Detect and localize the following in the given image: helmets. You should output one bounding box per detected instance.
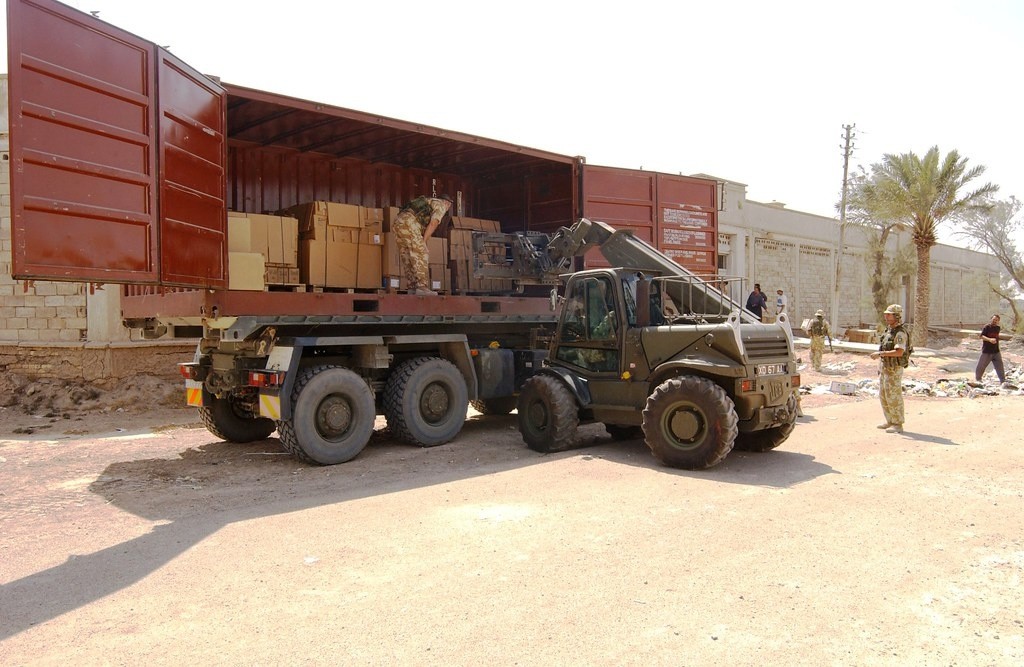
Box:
[883,304,903,314]
[814,309,826,317]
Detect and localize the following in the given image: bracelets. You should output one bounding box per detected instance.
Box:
[878,351,883,357]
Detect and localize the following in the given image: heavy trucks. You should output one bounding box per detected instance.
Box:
[6,0,719,466]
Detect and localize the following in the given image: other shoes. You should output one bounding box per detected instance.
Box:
[877,422,892,429]
[886,423,903,433]
[1001,379,1013,385]
[408,288,416,295]
[416,286,438,296]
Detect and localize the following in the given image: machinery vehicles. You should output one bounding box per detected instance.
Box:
[471,217,801,470]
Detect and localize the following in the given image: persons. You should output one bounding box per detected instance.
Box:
[805,309,834,371]
[723,279,728,296]
[869,304,910,433]
[392,193,455,295]
[581,310,616,363]
[745,284,769,322]
[975,315,1007,384]
[774,288,787,322]
